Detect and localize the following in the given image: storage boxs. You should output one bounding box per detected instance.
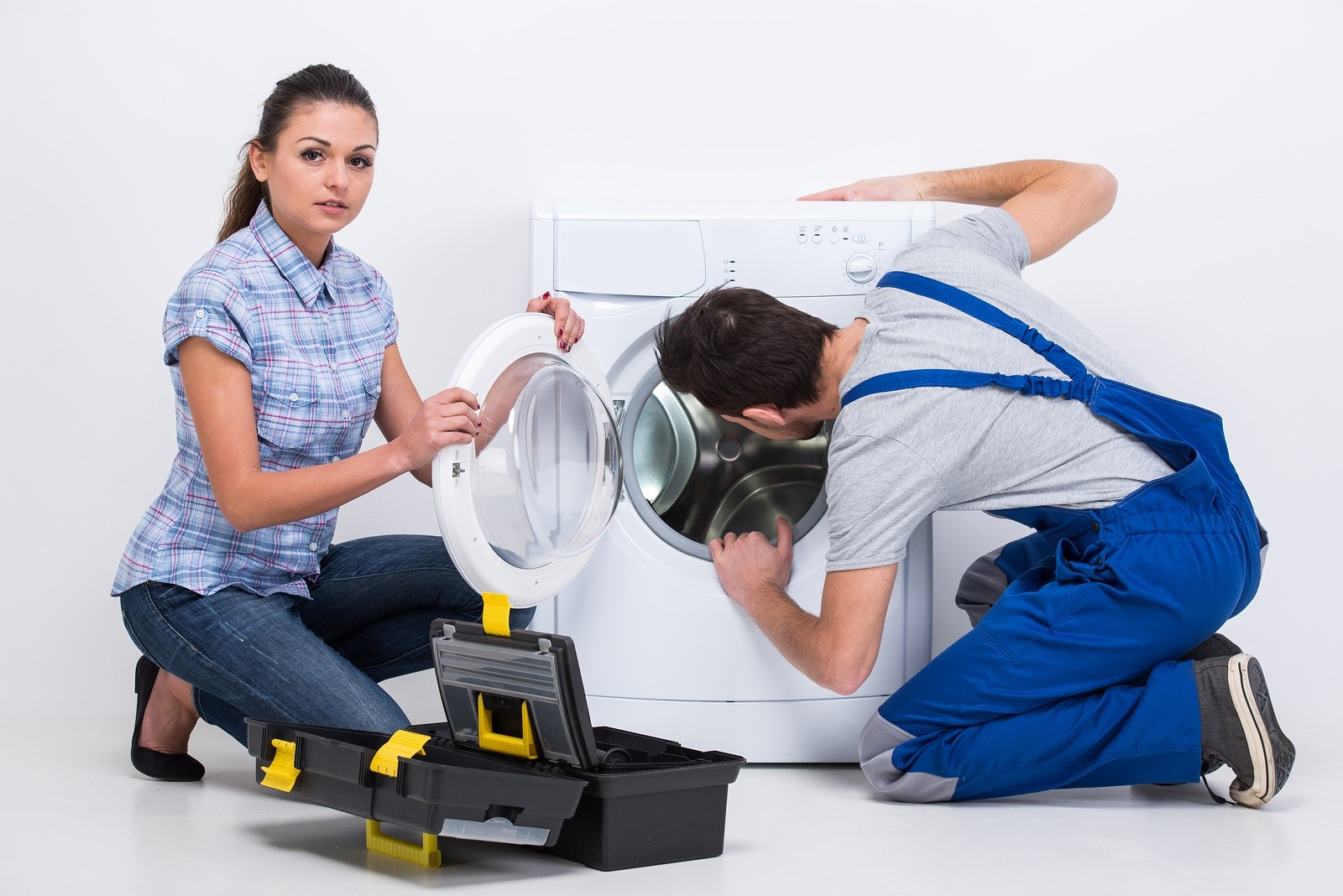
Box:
[244,591,746,872]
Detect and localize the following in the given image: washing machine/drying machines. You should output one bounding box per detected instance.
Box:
[428,204,934,770]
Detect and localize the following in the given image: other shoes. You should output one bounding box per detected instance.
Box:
[130,652,206,783]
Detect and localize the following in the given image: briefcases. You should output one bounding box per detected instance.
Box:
[243,615,747,872]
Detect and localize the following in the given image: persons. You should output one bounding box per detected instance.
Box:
[653,157,1295,808]
[112,66,584,787]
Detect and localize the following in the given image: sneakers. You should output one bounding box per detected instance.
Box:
[1196,633,1295,807]
